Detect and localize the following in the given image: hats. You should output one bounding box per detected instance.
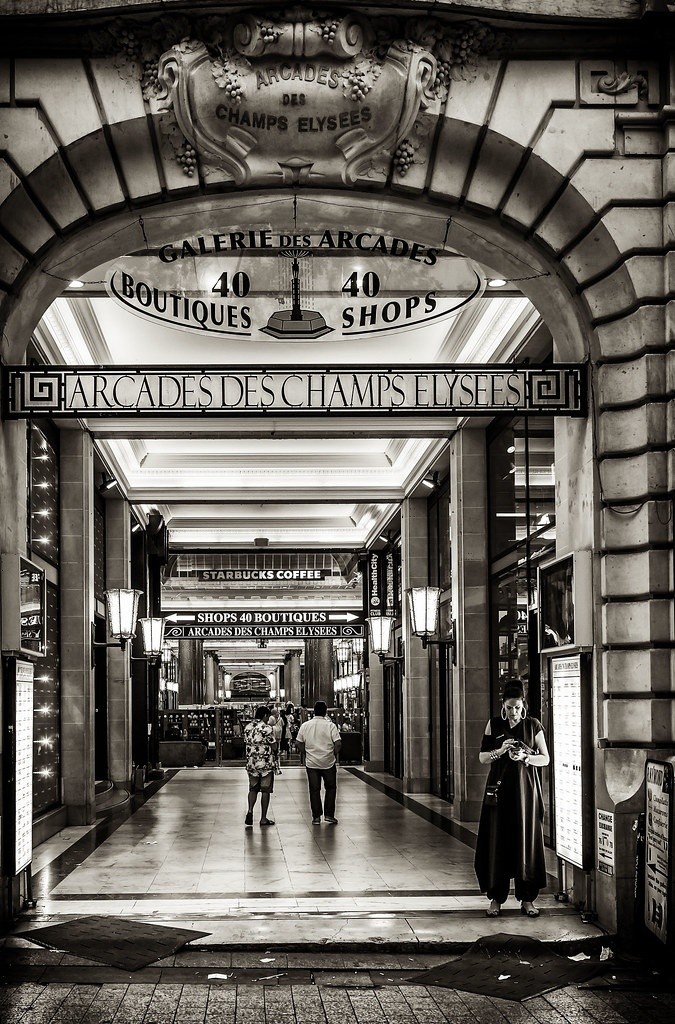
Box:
[501,680,524,699]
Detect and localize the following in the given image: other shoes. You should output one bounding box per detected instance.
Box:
[487,905,500,917]
[521,902,540,918]
[312,817,321,825]
[324,816,338,824]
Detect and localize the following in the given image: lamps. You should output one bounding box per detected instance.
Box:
[91,589,145,670]
[130,617,168,678]
[404,586,457,667]
[365,616,405,677]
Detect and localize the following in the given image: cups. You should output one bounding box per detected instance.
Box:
[156,761,161,770]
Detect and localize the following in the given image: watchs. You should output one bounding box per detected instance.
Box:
[524,753,530,764]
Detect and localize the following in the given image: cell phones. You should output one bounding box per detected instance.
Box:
[512,741,519,752]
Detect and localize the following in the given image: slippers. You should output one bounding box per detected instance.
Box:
[245,811,253,825]
[260,818,274,826]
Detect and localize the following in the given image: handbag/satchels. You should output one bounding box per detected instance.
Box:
[515,741,537,767]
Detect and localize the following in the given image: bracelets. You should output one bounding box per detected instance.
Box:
[489,749,501,761]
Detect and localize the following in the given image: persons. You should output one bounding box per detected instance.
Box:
[475,681,551,917]
[268,703,296,760]
[295,701,343,825]
[243,706,279,825]
